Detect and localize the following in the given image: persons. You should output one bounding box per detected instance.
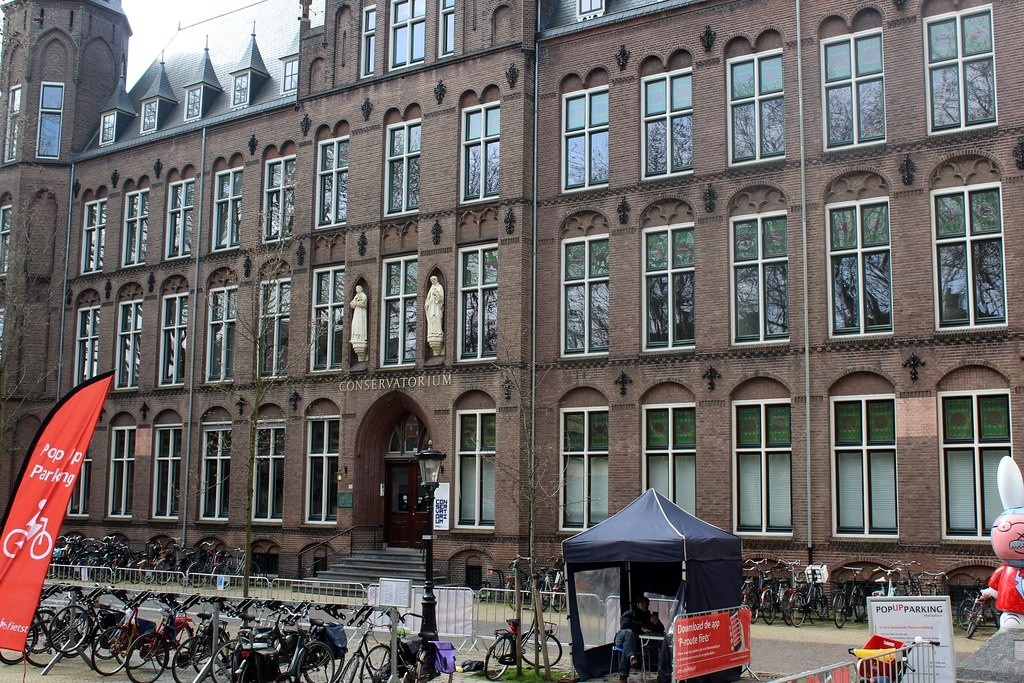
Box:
[349,284,370,344]
[613,597,666,683]
[424,275,445,338]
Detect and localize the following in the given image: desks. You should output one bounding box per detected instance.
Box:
[639,633,664,683]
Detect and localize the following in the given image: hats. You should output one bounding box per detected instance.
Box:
[636,597,650,604]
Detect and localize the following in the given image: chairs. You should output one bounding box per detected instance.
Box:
[610,646,650,678]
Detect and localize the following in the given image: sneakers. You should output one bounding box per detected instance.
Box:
[619,677,627,683]
[629,655,639,669]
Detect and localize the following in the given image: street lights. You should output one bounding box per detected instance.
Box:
[414,439,447,642]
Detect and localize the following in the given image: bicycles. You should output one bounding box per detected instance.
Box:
[0,582,453,683]
[849,638,940,683]
[485,608,562,680]
[741,558,949,629]
[958,576,990,639]
[505,553,566,612]
[47,536,259,589]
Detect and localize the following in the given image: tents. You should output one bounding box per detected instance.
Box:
[561,488,744,683]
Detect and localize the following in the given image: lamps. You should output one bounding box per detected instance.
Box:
[335,466,346,482]
